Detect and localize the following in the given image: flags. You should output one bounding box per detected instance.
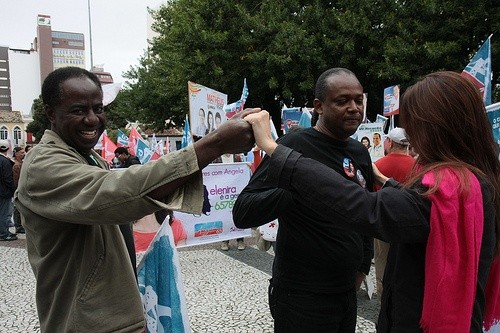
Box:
[482,102,500,151]
[100,114,195,166]
[226,80,249,118]
[460,37,492,106]
[298,110,312,128]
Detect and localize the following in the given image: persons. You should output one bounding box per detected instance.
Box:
[114,146,141,167]
[215,151,246,250]
[132,207,187,254]
[244,70,500,333]
[205,110,215,135]
[192,107,206,137]
[360,127,420,282]
[214,111,223,130]
[230,65,377,333]
[389,85,399,112]
[0,145,32,241]
[12,65,264,333]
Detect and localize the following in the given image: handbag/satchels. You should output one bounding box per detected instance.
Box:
[254,227,273,251]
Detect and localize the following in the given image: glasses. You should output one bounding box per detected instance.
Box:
[116,154,121,159]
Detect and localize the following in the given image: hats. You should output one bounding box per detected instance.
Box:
[13,147,23,157]
[0,140,9,151]
[385,127,409,145]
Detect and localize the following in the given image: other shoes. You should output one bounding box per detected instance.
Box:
[238,242,245,249]
[0,231,18,241]
[16,226,25,233]
[222,240,229,251]
